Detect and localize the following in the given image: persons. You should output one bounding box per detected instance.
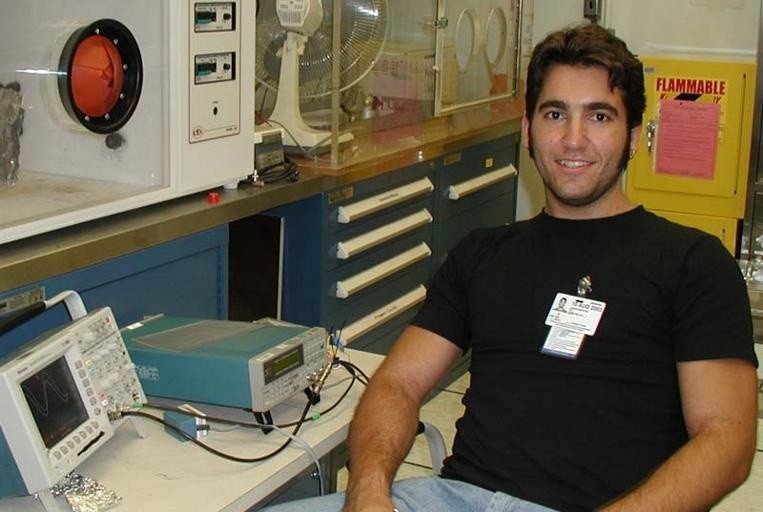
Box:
[554,297,569,312]
[256,20,762,512]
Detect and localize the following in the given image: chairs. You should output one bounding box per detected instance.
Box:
[346,421,448,486]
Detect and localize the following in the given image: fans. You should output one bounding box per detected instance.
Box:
[255,0,389,157]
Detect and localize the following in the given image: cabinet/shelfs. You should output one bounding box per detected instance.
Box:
[320,128,521,349]
[627,45,757,259]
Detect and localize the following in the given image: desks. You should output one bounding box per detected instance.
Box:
[0,347,390,512]
